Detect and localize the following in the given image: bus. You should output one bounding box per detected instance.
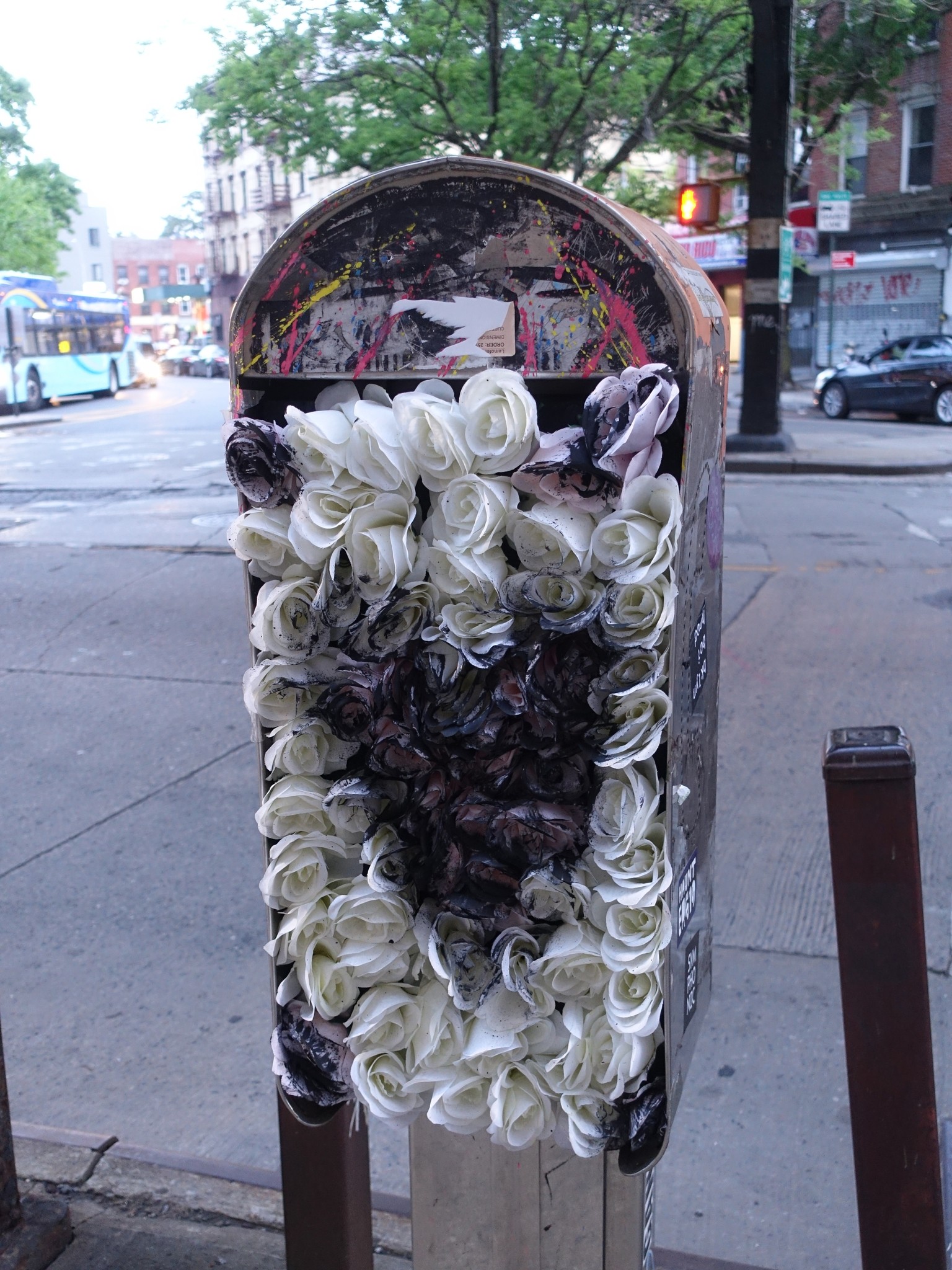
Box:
[0,272,137,413]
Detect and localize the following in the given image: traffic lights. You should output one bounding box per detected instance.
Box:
[676,180,721,226]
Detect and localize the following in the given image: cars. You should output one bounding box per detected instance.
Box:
[155,345,201,375]
[812,334,952,427]
[189,344,229,376]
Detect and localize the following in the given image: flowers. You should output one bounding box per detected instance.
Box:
[210,351,697,1160]
